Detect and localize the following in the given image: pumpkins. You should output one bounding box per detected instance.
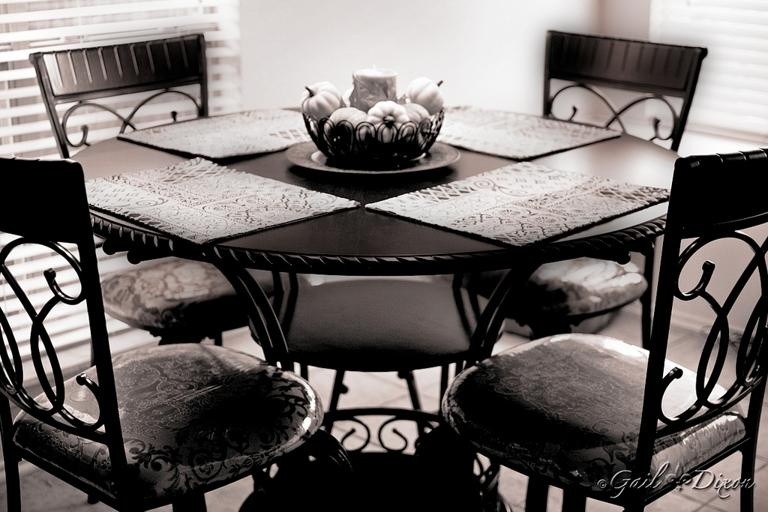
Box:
[301,78,444,149]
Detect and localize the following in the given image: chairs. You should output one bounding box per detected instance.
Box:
[0,31,768,512]
